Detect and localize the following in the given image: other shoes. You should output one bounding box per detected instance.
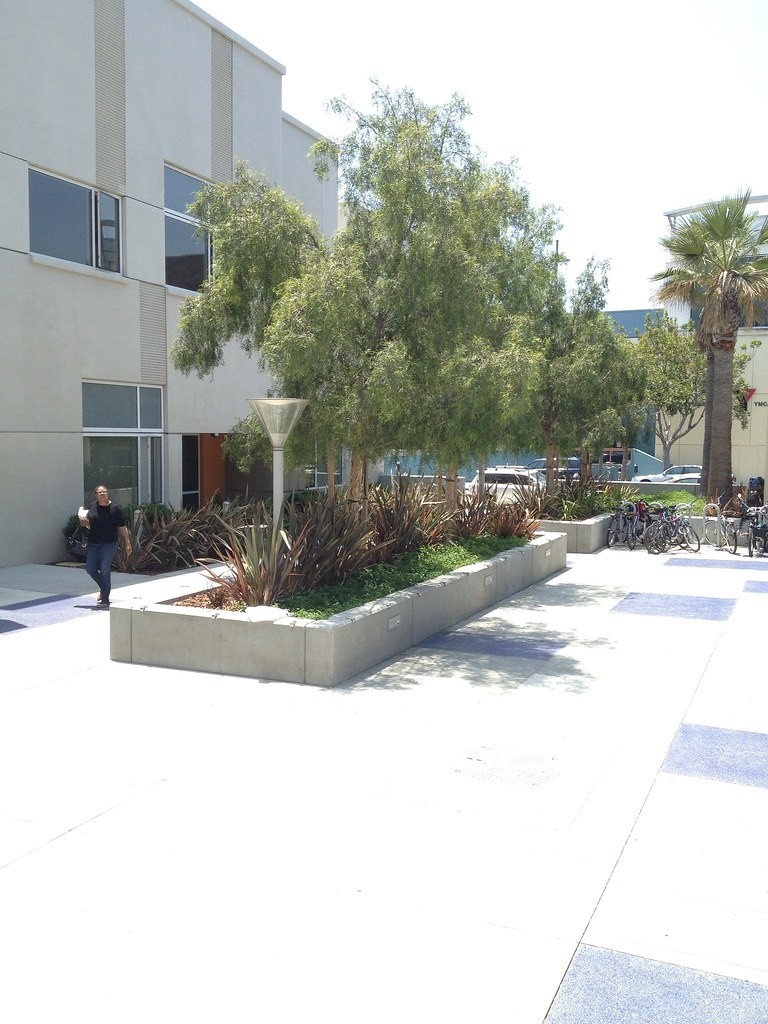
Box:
[97,603,110,608]
[97,591,102,601]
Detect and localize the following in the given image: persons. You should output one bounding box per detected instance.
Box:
[80,485,132,608]
[746,477,765,507]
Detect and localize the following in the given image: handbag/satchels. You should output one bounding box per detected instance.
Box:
[66,526,89,556]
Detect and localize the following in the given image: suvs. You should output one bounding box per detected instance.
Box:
[467,465,548,507]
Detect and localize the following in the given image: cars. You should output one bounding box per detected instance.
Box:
[526,457,583,479]
[605,500,768,558]
[631,464,737,484]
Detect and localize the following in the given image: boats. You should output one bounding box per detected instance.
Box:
[247,396,310,556]
